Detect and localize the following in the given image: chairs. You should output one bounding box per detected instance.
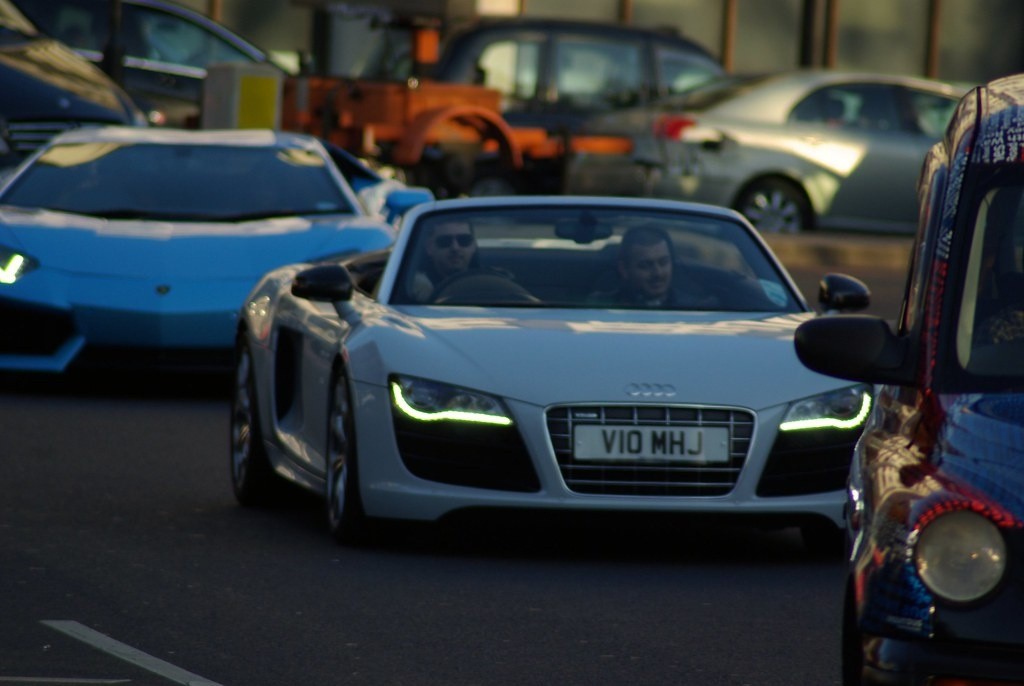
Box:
[471,242,774,310]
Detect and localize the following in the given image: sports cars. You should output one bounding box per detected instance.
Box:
[229,196,878,555]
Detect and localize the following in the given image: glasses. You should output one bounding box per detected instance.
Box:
[431,233,475,248]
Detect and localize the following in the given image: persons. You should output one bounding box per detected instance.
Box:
[584,228,718,312]
[408,216,530,304]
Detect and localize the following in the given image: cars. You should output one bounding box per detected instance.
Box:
[0,123,435,374]
[353,17,748,197]
[15,0,302,122]
[1,0,153,174]
[565,66,985,240]
[792,73,1024,686]
